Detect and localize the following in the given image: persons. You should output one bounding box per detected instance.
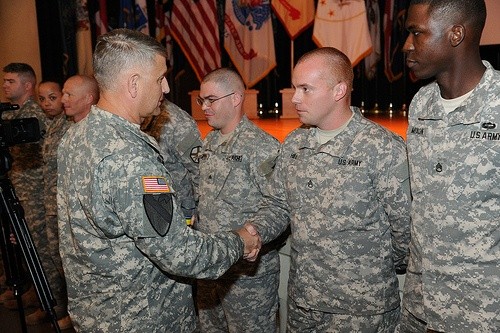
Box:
[191,67,281,333]
[142,98,202,229]
[56,28,261,333]
[0,62,99,333]
[244,47,412,333]
[398,0,500,333]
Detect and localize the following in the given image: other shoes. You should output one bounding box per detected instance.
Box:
[0,275,72,329]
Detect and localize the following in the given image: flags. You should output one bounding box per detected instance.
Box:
[73,0,422,95]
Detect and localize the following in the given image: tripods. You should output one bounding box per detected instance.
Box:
[0,149,61,333]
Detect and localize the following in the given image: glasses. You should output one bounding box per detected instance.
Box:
[196,92,234,106]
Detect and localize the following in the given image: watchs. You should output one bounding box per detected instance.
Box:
[228,231,244,252]
